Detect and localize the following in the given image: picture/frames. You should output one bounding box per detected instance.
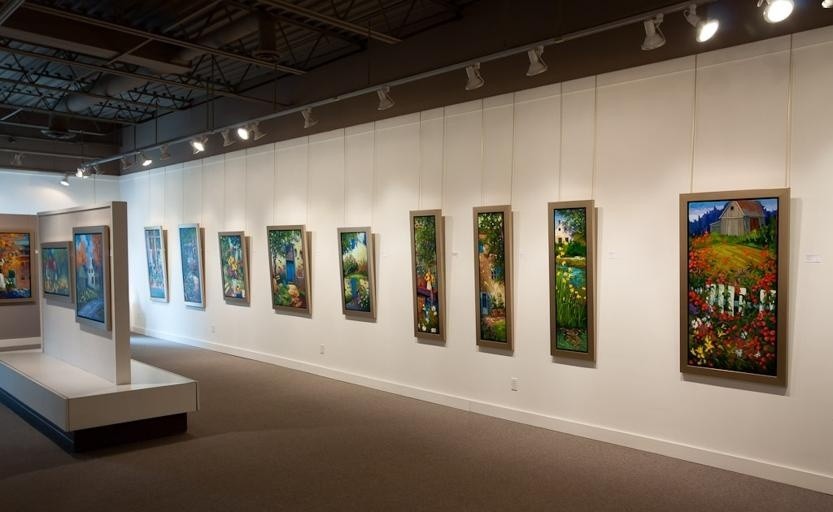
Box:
[143,225,377,320]
[409,200,598,362]
[0,225,109,330]
[681,186,790,388]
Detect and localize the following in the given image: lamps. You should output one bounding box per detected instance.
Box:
[640,0,833,54]
[60,140,172,190]
[187,118,265,155]
[377,83,394,113]
[300,105,317,131]
[459,45,549,92]
